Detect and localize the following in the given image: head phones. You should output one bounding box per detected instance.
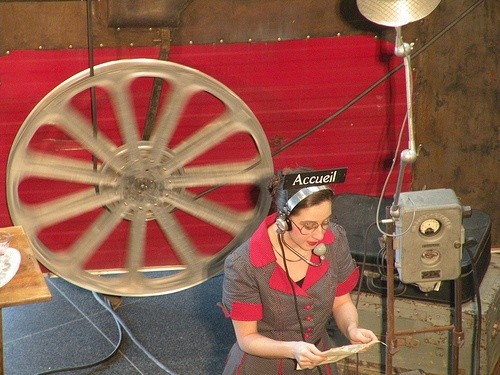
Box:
[276,184,334,234]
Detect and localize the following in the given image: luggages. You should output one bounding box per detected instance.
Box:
[331,193,492,304]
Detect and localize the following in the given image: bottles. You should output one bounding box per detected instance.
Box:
[0,230,11,268]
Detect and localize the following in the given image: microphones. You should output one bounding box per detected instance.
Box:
[281,234,326,267]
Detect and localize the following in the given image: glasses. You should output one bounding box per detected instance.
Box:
[286,216,336,235]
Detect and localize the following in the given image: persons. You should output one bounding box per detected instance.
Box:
[221,166,380,375]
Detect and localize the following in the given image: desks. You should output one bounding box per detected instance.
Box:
[0,226,52,375]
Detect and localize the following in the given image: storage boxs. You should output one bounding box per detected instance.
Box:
[326,253,500,375]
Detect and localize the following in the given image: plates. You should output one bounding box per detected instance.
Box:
[0,248,21,287]
